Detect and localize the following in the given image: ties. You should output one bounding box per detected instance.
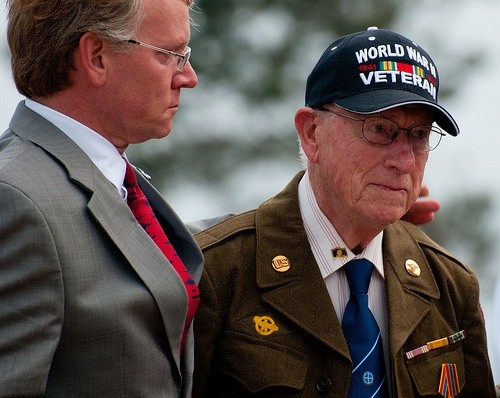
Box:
[341,259,390,398]
[123,161,200,355]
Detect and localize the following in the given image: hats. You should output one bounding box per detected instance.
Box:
[305,26,460,136]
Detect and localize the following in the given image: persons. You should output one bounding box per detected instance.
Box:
[0,1,440,398]
[191,26,498,398]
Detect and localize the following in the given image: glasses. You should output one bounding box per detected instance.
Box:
[311,105,446,152]
[124,39,192,70]
